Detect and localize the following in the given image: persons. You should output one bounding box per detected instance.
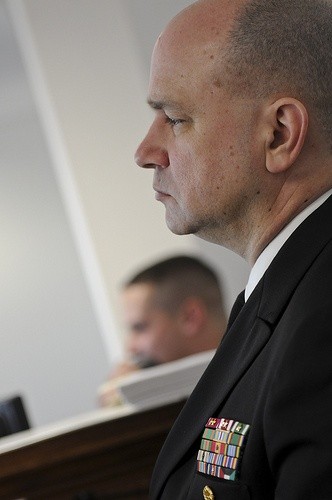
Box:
[121,254,230,366]
[130,0,331,499]
